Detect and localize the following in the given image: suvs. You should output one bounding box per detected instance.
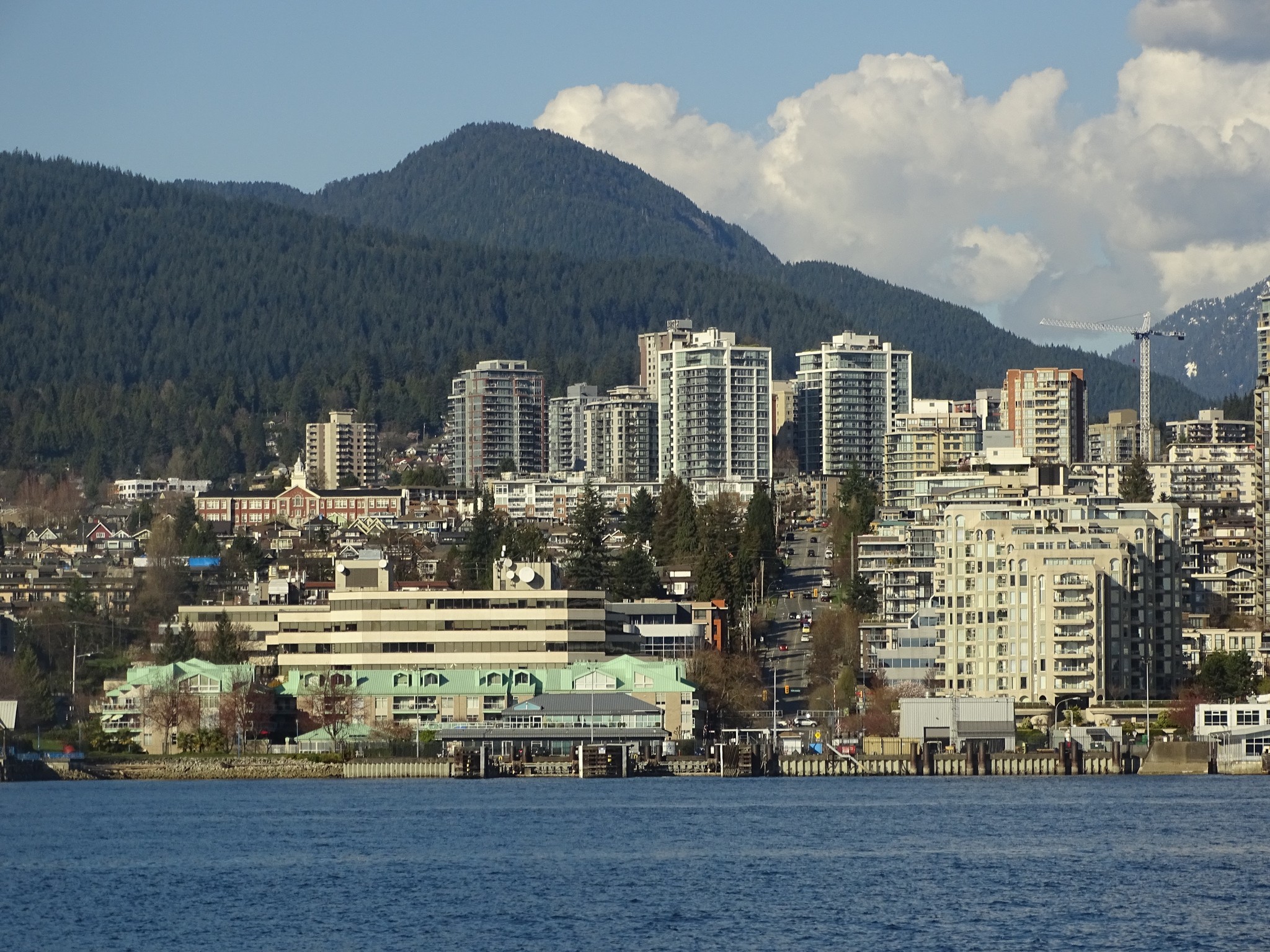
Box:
[760,717,787,728]
[535,747,549,757]
[808,550,815,557]
[811,537,817,543]
[786,533,795,541]
[794,718,817,728]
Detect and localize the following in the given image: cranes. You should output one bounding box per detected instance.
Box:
[1039,311,1185,461]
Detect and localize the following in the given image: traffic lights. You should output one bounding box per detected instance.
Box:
[785,685,789,694]
[814,589,817,597]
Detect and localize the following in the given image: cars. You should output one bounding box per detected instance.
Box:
[806,517,813,523]
[801,635,810,642]
[779,643,788,650]
[822,522,828,528]
[802,592,812,599]
[821,546,833,601]
[787,549,794,555]
[790,610,813,632]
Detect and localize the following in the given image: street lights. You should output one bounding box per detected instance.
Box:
[1140,656,1152,750]
[767,580,782,597]
[815,698,834,740]
[72,645,91,711]
[816,676,833,687]
[768,658,782,754]
[413,666,423,756]
[587,659,599,743]
[1055,697,1080,730]
